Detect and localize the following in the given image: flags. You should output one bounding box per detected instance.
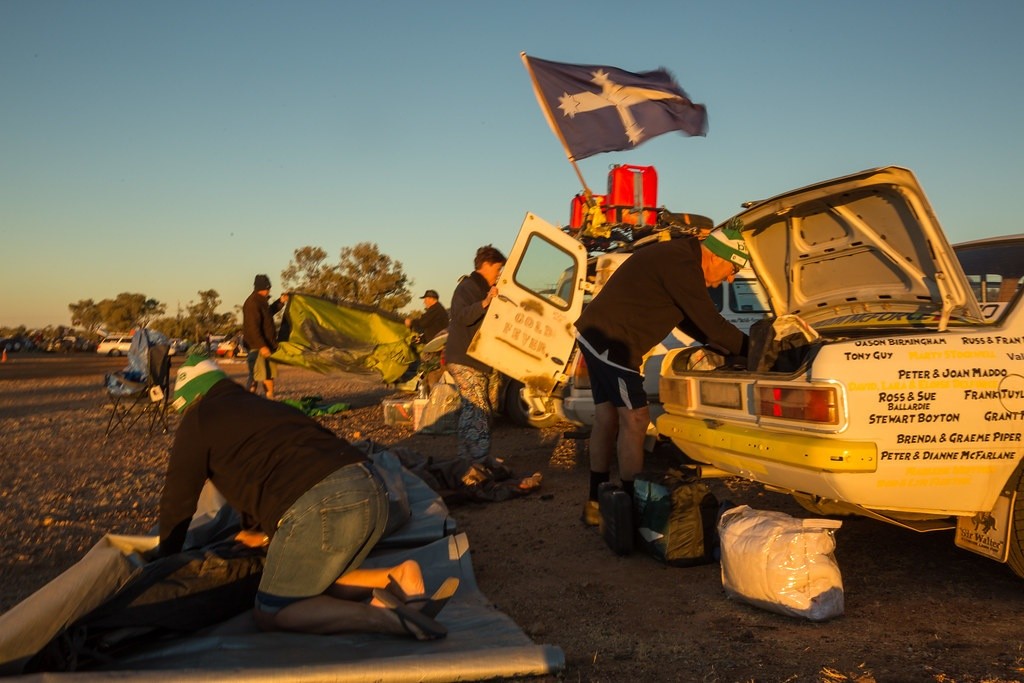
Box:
[526,56,710,160]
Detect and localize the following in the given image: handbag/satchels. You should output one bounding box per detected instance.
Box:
[636,464,719,567]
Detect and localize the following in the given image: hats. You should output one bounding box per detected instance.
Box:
[702,217,750,268]
[419,290,438,299]
[174,343,227,413]
[254,274,270,290]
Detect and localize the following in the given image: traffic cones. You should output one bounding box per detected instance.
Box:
[0,348,9,363]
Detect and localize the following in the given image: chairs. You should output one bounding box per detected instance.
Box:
[103,329,171,438]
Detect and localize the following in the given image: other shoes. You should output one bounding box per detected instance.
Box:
[584,502,603,525]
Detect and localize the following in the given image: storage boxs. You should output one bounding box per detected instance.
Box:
[606,165,657,226]
[382,399,414,427]
[413,399,429,430]
[570,194,608,228]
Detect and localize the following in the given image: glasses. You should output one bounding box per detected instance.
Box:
[733,263,739,274]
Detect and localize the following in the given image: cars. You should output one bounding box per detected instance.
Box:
[464,208,775,466]
[215,329,250,359]
[0,338,26,352]
[94,328,182,357]
[652,163,1024,589]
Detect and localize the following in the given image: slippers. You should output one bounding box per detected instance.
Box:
[373,589,447,639]
[404,576,460,618]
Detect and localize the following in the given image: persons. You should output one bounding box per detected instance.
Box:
[571,227,752,529]
[16,324,243,357]
[242,274,288,398]
[147,355,460,641]
[405,290,450,392]
[443,242,505,459]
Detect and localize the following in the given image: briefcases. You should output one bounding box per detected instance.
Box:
[598,482,633,555]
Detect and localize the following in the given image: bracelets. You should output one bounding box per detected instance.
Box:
[261,534,269,545]
